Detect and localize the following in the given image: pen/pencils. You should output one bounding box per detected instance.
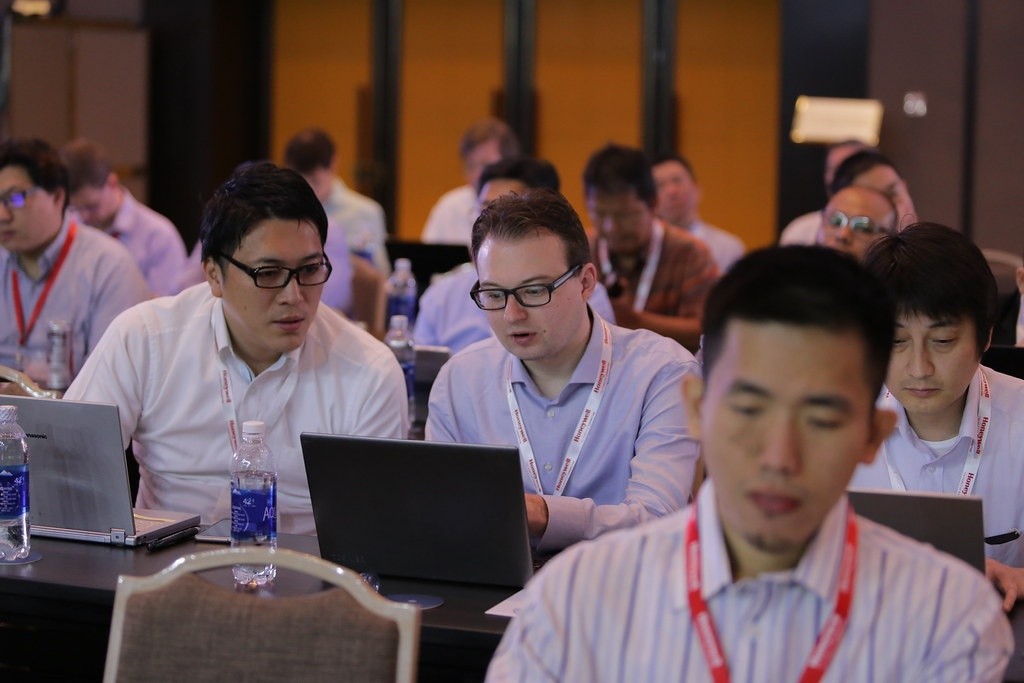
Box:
[146,526,201,553]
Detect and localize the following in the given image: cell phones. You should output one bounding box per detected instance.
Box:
[194,517,232,542]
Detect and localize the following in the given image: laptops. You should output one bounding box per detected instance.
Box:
[299,433,551,587]
[384,241,472,294]
[389,343,454,387]
[845,490,986,575]
[0,393,200,546]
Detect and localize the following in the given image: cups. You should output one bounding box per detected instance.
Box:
[44,320,72,389]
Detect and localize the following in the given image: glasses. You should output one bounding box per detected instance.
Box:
[823,211,890,243]
[471,262,587,310]
[0,184,42,209]
[223,252,332,289]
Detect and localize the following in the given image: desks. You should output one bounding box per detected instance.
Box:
[0,514,568,683]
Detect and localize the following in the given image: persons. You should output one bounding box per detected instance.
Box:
[778,139,918,261]
[171,222,352,309]
[484,245,1016,683]
[845,222,1024,611]
[650,150,745,273]
[59,138,186,298]
[424,187,703,554]
[61,162,410,536]
[420,116,521,245]
[582,145,722,354]
[410,157,617,356]
[0,138,148,399]
[284,127,389,307]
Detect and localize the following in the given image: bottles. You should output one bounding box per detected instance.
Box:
[229,420,276,586]
[383,258,417,336]
[385,314,417,431]
[0,404,31,561]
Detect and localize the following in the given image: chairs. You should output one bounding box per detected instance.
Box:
[104,545,420,683]
